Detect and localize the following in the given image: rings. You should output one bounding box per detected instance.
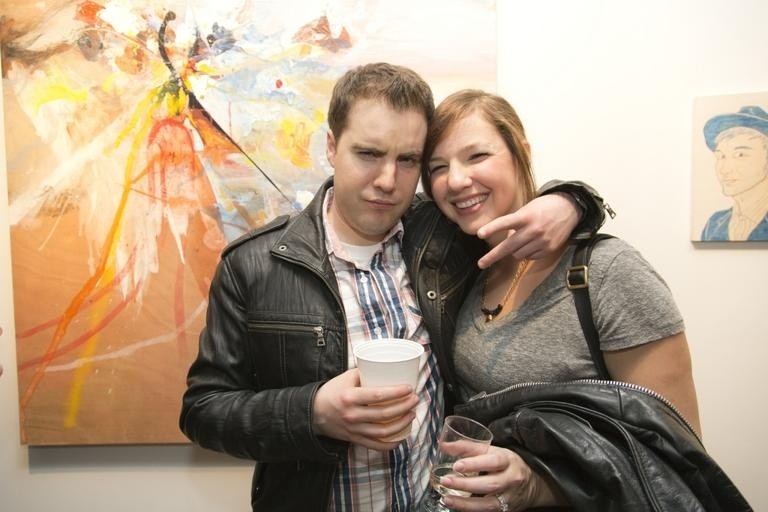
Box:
[494,492,511,511]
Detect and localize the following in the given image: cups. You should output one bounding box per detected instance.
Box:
[350,337,427,444]
[414,414,494,501]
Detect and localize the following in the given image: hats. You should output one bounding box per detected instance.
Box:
[703,105,768,154]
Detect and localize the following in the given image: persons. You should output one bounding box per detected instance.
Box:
[701,105,767,242]
[176,62,617,511]
[419,87,701,512]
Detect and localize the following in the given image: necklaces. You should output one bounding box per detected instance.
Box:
[479,258,530,321]
